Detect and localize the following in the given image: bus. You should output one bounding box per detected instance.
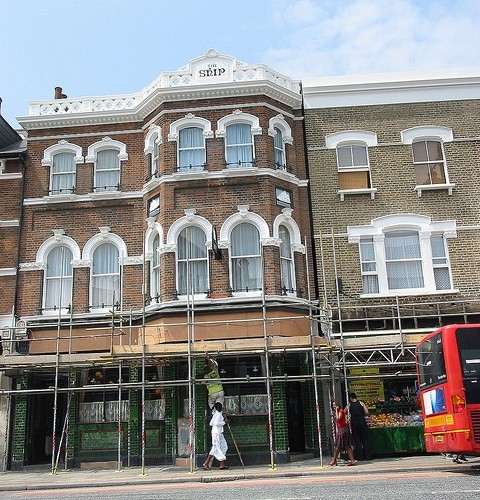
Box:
[415,324,480,458]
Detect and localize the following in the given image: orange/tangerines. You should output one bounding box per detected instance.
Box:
[371,414,390,425]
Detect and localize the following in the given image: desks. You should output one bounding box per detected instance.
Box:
[368,426,425,454]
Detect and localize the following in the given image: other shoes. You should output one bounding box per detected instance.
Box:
[328,459,337,466]
[446,455,453,459]
[347,462,357,466]
[458,457,468,462]
[453,459,462,464]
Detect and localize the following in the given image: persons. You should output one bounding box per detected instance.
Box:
[344,392,369,461]
[203,402,231,470]
[328,400,357,466]
[202,359,225,414]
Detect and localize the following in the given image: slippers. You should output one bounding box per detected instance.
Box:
[203,466,210,471]
[220,466,228,469]
